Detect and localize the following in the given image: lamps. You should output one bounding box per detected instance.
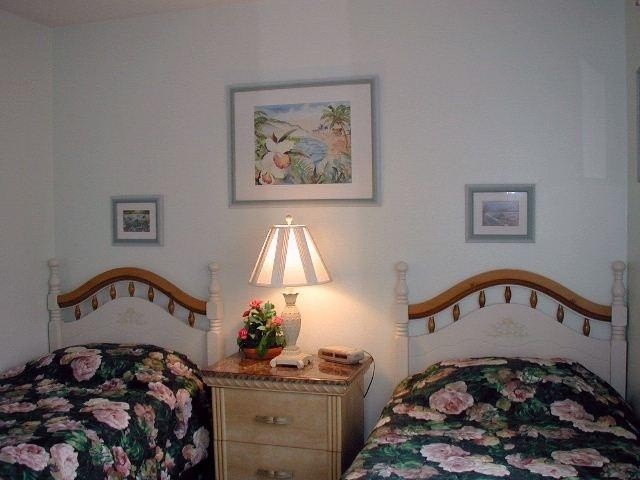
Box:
[246,213,332,373]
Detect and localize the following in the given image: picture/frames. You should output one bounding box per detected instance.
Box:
[221,73,381,209]
[108,190,165,250]
[462,182,536,246]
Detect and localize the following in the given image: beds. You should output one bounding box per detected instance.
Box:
[0,256,223,480]
[338,252,638,479]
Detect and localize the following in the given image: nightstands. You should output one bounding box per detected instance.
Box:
[199,349,377,479]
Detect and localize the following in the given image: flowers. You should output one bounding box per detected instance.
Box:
[235,299,285,356]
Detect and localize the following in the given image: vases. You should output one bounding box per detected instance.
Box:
[242,347,283,363]
